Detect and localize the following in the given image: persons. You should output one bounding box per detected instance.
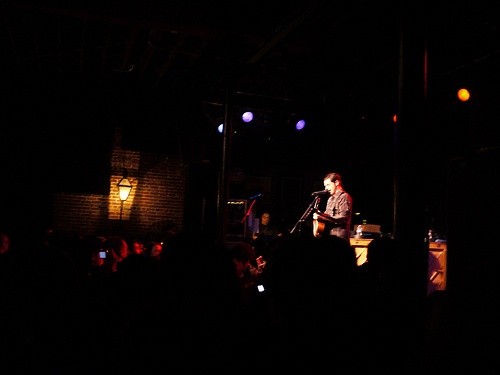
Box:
[0,198,500,375]
[314,172,354,244]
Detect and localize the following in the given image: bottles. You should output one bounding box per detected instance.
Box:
[362,208,368,225]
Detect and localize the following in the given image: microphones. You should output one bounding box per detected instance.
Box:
[250,194,261,200]
[312,190,328,195]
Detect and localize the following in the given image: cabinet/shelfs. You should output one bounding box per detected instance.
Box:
[222,199,251,248]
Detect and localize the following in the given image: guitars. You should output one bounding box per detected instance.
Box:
[312,196,325,238]
